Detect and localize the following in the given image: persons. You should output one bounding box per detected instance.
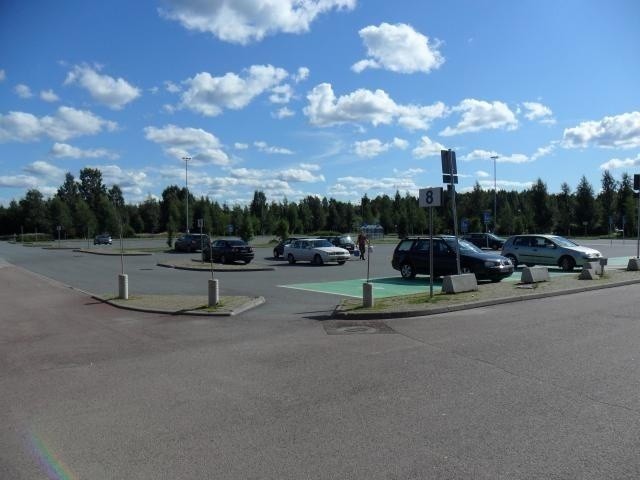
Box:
[355,230,370,260]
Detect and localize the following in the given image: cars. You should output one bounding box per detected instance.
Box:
[175,233,210,252]
[391,232,602,283]
[203,238,254,264]
[93,234,112,245]
[273,235,355,266]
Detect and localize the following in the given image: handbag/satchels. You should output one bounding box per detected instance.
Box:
[368,246,374,253]
[354,249,359,256]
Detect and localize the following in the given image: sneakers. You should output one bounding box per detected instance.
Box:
[360,255,366,260]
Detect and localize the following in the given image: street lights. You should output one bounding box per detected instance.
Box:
[490,156,499,224]
[181,155,191,233]
